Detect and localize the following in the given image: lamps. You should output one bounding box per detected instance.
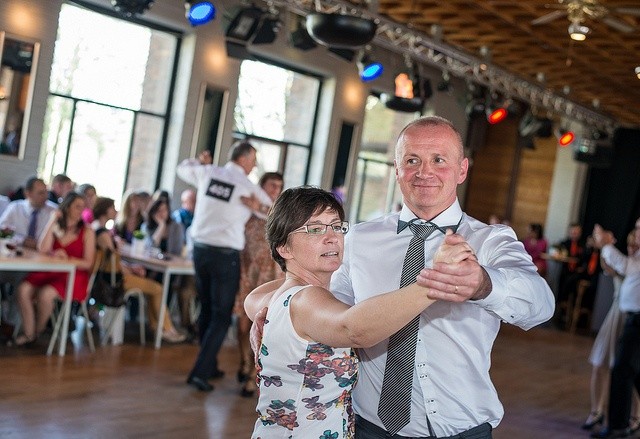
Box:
[219,0,283,46]
[183,0,216,27]
[110,0,155,18]
[290,12,606,148]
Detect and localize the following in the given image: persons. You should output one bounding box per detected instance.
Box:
[176,140,272,394]
[8,193,97,348]
[91,198,188,345]
[234,171,284,400]
[0,116,640,439]
[243,117,555,439]
[249,185,476,439]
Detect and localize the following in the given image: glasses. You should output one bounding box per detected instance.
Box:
[287,221,350,235]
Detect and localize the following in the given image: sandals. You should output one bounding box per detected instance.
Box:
[8,334,37,348]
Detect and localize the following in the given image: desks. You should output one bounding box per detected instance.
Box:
[122,255,197,350]
[0,256,76,356]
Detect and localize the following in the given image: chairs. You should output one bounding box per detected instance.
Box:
[42,251,146,357]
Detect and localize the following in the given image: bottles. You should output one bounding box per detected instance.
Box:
[131,230,145,255]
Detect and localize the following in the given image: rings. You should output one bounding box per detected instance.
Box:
[455,285,458,294]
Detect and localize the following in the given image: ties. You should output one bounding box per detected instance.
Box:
[377,222,438,436]
[27,211,37,237]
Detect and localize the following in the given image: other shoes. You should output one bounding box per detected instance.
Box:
[186,377,214,392]
[588,429,627,439]
[242,388,254,397]
[163,328,186,343]
[206,371,225,379]
[237,372,251,383]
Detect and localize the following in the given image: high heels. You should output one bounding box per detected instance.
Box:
[580,411,605,431]
[626,417,640,433]
